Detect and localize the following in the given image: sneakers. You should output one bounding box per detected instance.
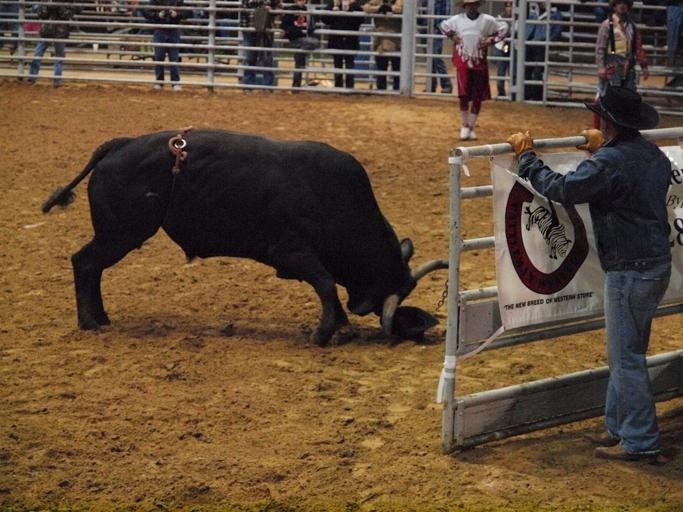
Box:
[584,432,619,446]
[153,85,182,92]
[459,127,476,140]
[441,88,453,93]
[595,445,658,460]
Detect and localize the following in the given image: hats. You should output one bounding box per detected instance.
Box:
[584,86,659,130]
[379,306,439,340]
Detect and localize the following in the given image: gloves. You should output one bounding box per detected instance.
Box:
[506,131,533,154]
[378,5,391,13]
[577,129,604,152]
[479,36,494,49]
[453,35,461,44]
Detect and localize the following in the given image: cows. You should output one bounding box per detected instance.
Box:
[42,128,450,348]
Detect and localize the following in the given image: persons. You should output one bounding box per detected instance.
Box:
[506,85,673,463]
[140,1,186,91]
[436,0,510,141]
[416,1,452,93]
[493,0,564,101]
[361,1,404,90]
[181,1,320,92]
[594,1,651,95]
[321,1,365,89]
[2,2,79,88]
